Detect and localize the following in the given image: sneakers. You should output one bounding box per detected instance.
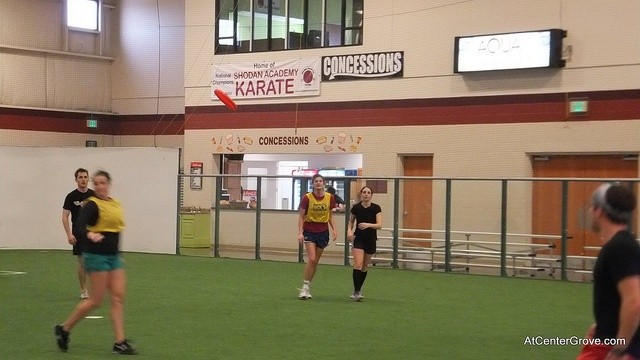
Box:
[350,293,355,299]
[80,289,90,299]
[112,340,137,354]
[355,292,364,301]
[296,288,312,299]
[55,325,70,352]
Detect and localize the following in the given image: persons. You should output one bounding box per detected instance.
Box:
[327,187,344,204]
[576,182,640,360]
[62,167,94,298]
[298,174,337,299]
[53,170,138,355]
[347,185,382,302]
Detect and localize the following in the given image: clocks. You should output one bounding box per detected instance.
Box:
[190,161,203,188]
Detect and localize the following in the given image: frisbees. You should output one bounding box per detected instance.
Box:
[214,88,238,111]
